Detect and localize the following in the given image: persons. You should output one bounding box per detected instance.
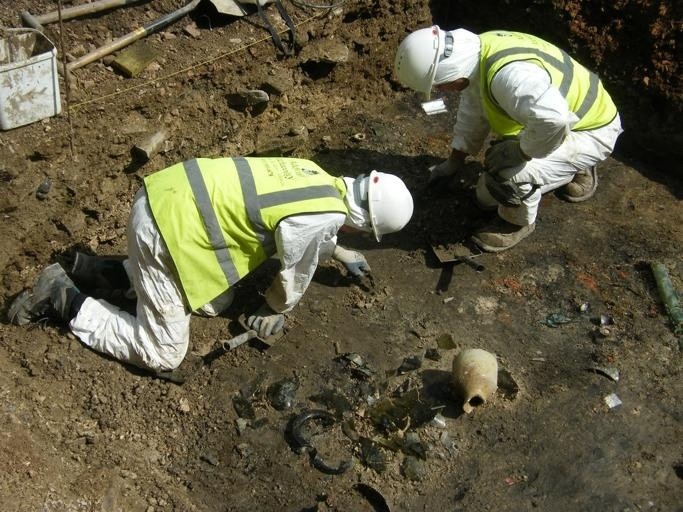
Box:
[392,20,625,257]
[2,153,414,374]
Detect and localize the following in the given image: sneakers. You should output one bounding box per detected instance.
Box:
[7,248,103,327]
[471,214,536,252]
[563,165,599,202]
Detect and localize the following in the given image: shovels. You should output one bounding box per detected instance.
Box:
[427,238,485,271]
[64,0,248,74]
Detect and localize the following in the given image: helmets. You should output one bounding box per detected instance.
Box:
[368,170,414,243]
[395,25,442,101]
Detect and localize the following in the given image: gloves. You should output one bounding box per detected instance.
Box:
[483,140,530,174]
[248,302,287,338]
[428,157,463,181]
[334,245,375,288]
